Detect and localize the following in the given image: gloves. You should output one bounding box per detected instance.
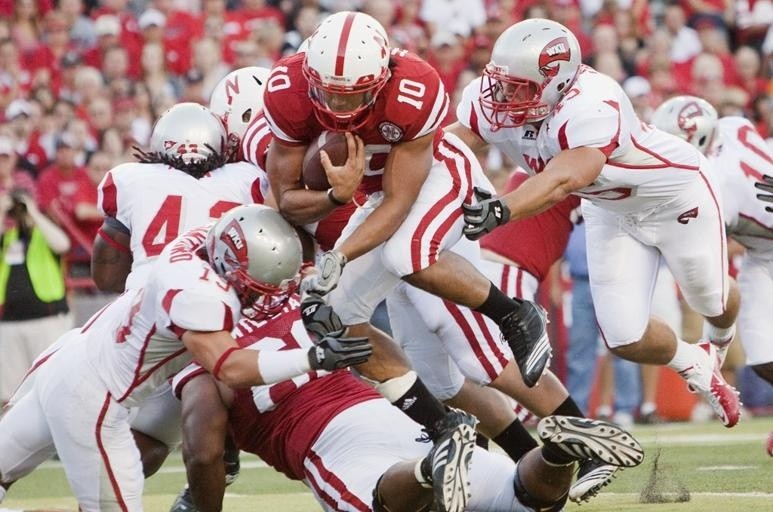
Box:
[460,184,510,241]
[753,172,773,214]
[301,249,348,297]
[308,329,374,372]
[297,297,343,347]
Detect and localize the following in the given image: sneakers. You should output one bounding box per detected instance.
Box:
[496,292,553,389]
[421,410,479,512]
[567,452,624,501]
[686,338,742,428]
[536,413,645,472]
[683,328,738,394]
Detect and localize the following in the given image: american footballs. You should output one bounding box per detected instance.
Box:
[303,130,348,191]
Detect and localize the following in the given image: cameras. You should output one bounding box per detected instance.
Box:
[10,196,27,219]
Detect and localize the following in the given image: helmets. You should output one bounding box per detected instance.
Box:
[206,202,309,323]
[148,101,229,172]
[649,95,719,156]
[208,63,275,145]
[301,8,392,138]
[478,18,583,132]
